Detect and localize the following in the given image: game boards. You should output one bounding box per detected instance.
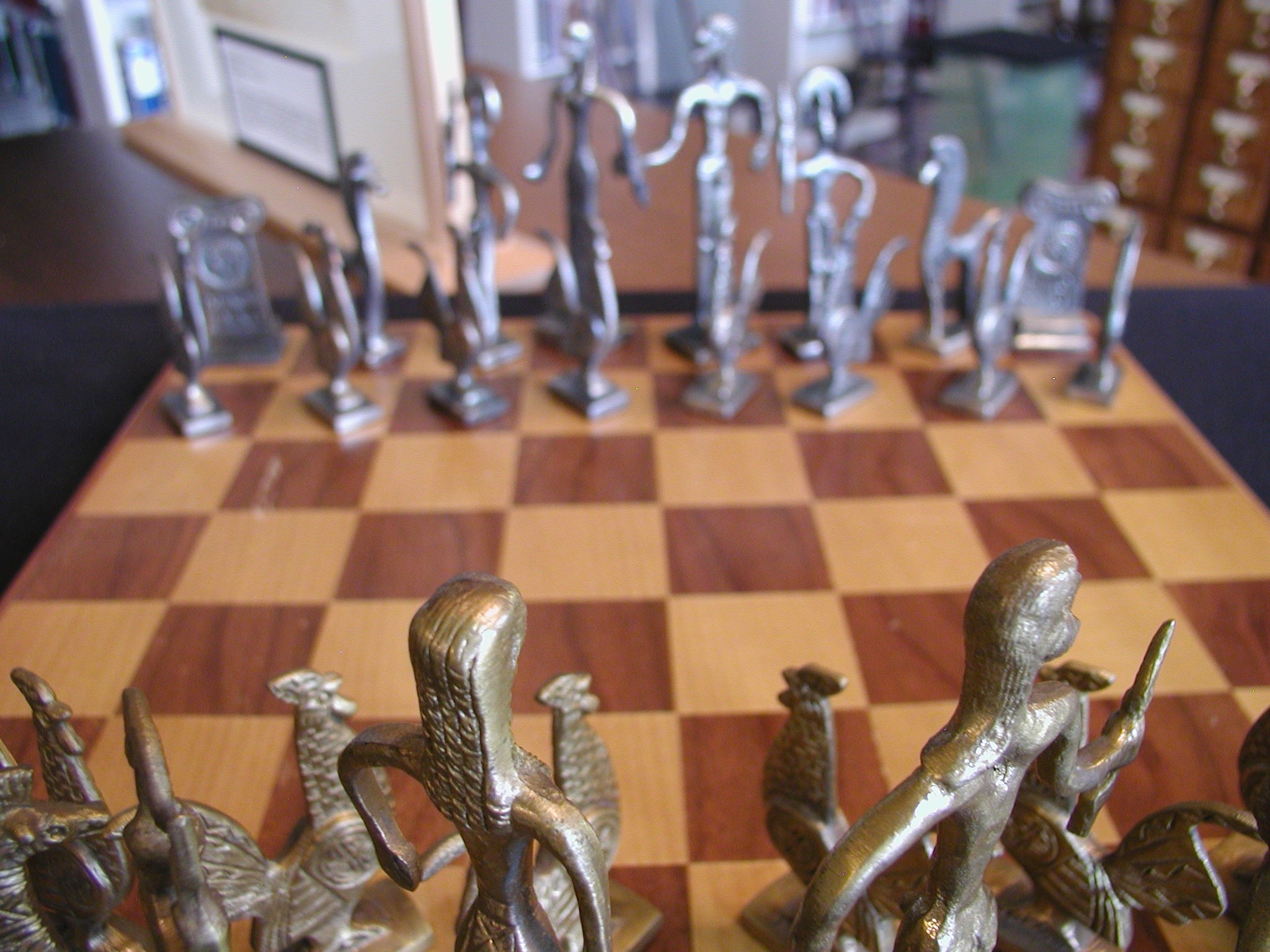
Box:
[0,304,1270,952]
